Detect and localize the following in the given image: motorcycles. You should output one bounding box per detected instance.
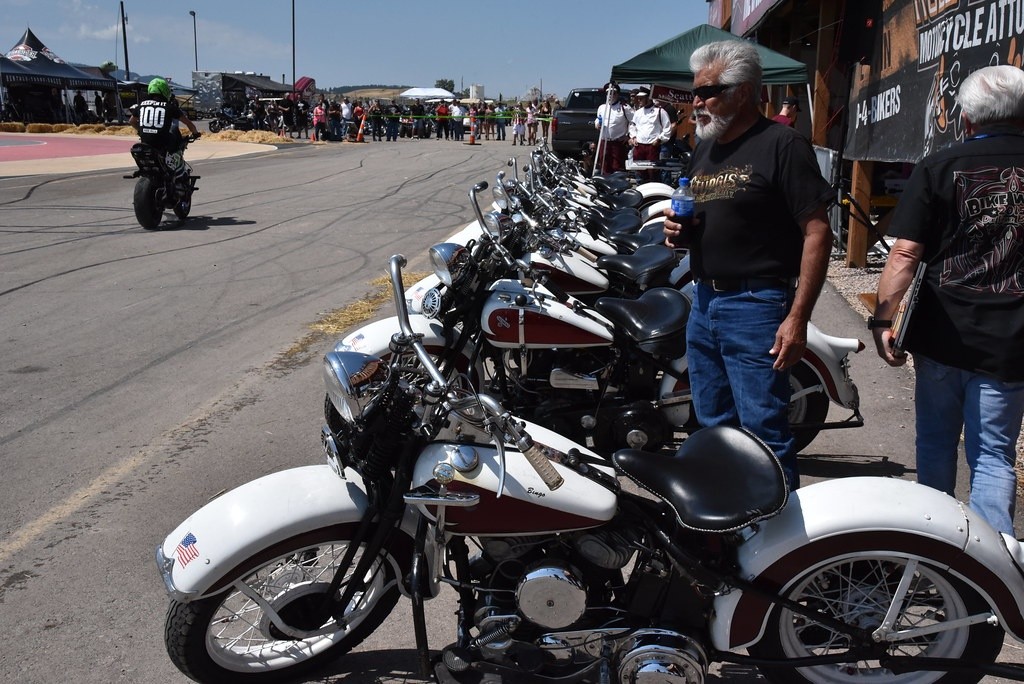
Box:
[208,104,255,134]
[154,128,1024,684]
[122,130,203,229]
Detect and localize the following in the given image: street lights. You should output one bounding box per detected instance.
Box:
[189,9,199,71]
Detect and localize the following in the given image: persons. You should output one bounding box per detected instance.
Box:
[128,78,199,153]
[663,39,833,492]
[629,86,672,184]
[74,91,89,117]
[658,97,678,153]
[630,89,642,110]
[171,93,179,125]
[772,96,802,129]
[869,65,1024,537]
[594,82,634,174]
[249,92,563,146]
[94,91,102,117]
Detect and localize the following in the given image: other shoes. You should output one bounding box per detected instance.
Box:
[386,139,390,141]
[393,139,396,141]
[296,136,301,139]
[521,144,523,145]
[306,137,310,139]
[290,136,294,139]
[523,139,527,141]
[502,139,505,140]
[436,137,439,140]
[379,139,381,141]
[496,138,500,140]
[513,144,516,145]
[373,139,377,141]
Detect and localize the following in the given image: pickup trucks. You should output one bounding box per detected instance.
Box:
[550,87,633,159]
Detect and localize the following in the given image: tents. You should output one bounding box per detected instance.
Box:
[592,24,815,174]
[401,87,455,98]
[0,27,127,124]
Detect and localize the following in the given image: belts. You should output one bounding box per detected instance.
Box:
[696,276,772,292]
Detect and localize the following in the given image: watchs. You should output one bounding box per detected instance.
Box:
[868,316,892,330]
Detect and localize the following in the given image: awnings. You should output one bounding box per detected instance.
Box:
[224,72,298,91]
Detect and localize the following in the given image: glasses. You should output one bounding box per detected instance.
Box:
[692,83,740,101]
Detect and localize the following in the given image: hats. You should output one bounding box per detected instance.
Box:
[604,83,621,94]
[636,86,650,96]
[782,97,802,112]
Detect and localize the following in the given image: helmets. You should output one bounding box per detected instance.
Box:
[148,78,171,101]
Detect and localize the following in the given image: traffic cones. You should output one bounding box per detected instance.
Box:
[310,132,315,142]
[280,131,285,138]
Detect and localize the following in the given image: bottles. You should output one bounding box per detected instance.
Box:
[668,176,695,245]
[598,115,602,128]
[628,150,633,165]
